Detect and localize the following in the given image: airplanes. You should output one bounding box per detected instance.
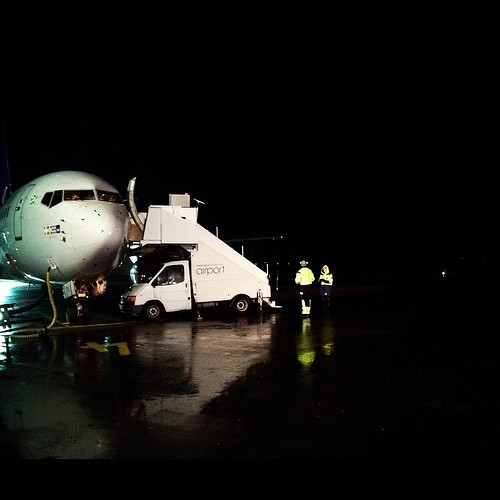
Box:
[0,169,151,326]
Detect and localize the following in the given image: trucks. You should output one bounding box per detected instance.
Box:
[117,191,284,321]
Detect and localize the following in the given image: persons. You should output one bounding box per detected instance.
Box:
[62,280,78,326]
[295,261,314,317]
[318,265,333,307]
